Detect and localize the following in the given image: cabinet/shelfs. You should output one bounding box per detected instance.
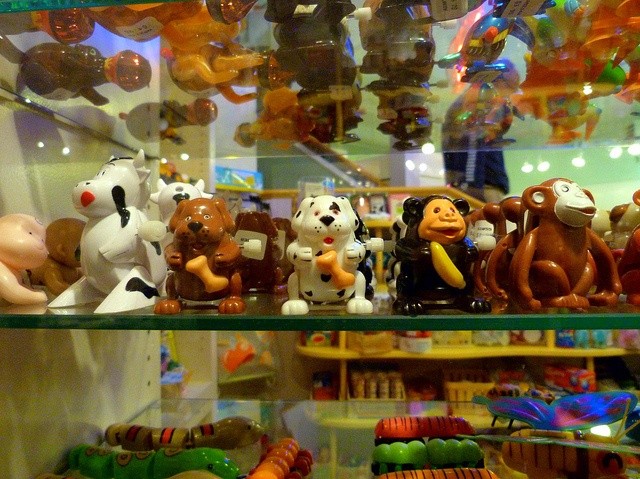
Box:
[0,0,640,478]
[294,331,640,478]
